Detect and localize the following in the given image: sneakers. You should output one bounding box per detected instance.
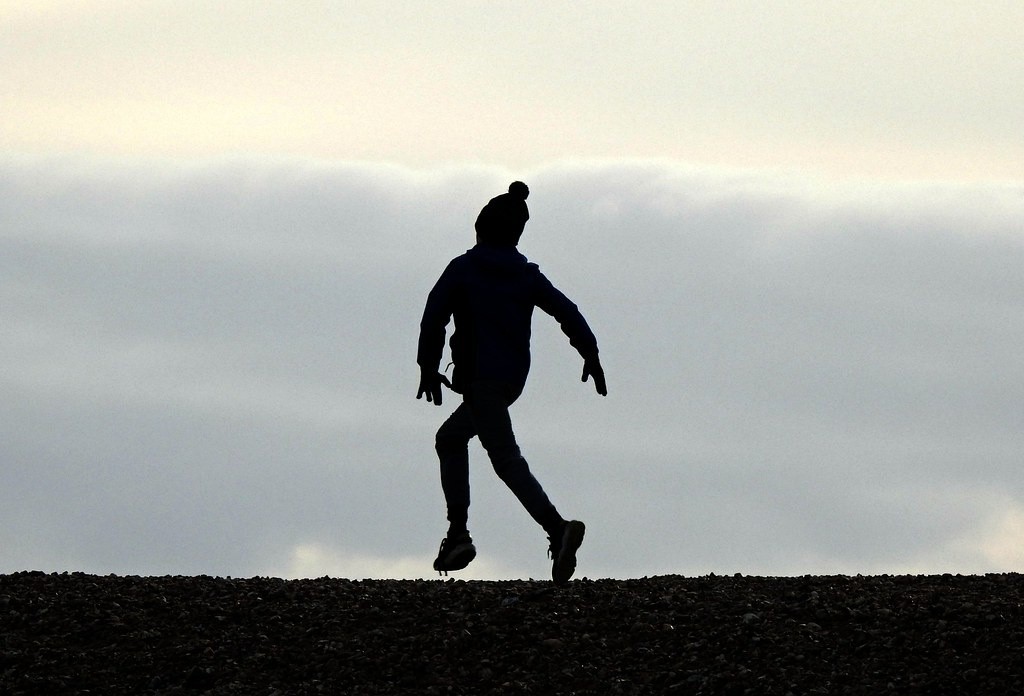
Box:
[551,520,586,583]
[432,535,477,576]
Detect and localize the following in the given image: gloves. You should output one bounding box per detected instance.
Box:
[416,367,452,406]
[581,357,607,396]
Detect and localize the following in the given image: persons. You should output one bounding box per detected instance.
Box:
[413,181,608,583]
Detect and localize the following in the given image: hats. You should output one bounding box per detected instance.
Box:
[474,182,531,246]
